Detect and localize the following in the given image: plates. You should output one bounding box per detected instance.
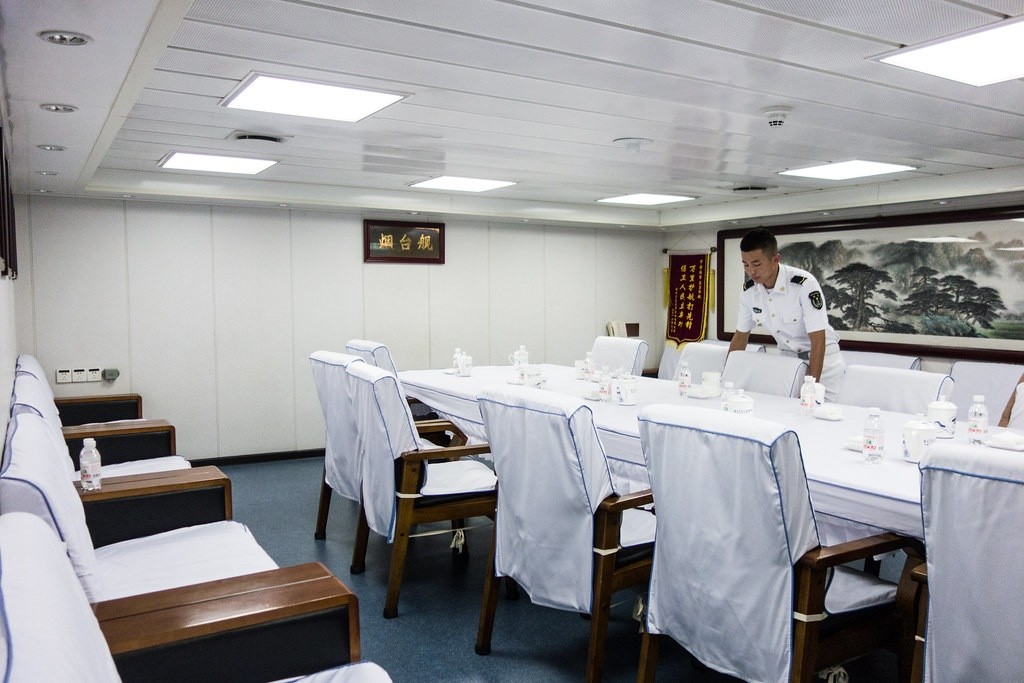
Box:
[687,386,721,398]
[981,430,1024,451]
[814,403,850,421]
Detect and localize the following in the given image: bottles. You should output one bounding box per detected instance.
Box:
[800,376,814,416]
[583,351,594,381]
[515,344,527,382]
[79,438,101,491]
[969,396,988,446]
[452,347,471,377]
[599,366,612,401]
[863,407,885,463]
[720,381,735,414]
[678,362,691,396]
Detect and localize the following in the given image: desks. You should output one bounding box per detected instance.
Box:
[391,365,1024,562]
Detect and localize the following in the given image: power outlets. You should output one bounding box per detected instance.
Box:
[57,369,72,383]
[87,368,102,382]
[73,368,86,383]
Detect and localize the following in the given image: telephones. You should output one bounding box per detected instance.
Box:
[606,321,628,337]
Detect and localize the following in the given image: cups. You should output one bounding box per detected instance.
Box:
[509,352,528,367]
[575,361,585,379]
[929,396,958,439]
[902,413,937,464]
[729,389,754,415]
[702,371,721,389]
[812,379,824,407]
[616,372,639,406]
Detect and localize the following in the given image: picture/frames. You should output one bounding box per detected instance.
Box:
[363,219,446,265]
[717,204,1024,368]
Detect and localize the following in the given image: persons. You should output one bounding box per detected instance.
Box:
[727,230,844,401]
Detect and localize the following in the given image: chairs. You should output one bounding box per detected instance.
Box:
[0,339,497,683]
[475,383,658,683]
[588,335,1024,683]
